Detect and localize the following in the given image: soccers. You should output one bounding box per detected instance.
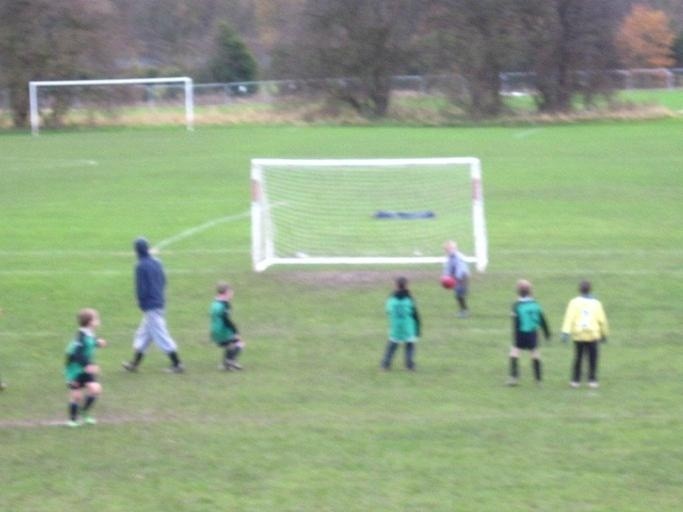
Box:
[440,276,456,290]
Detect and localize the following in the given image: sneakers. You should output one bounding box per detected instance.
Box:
[505,377,519,386]
[570,380,599,389]
[64,406,96,427]
[455,309,471,319]
[122,361,139,371]
[217,356,242,372]
[162,362,183,372]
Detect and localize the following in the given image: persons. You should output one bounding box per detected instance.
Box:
[59,308,110,429]
[117,237,185,376]
[205,282,246,372]
[558,279,609,390]
[440,238,472,318]
[502,277,552,390]
[378,273,423,372]
